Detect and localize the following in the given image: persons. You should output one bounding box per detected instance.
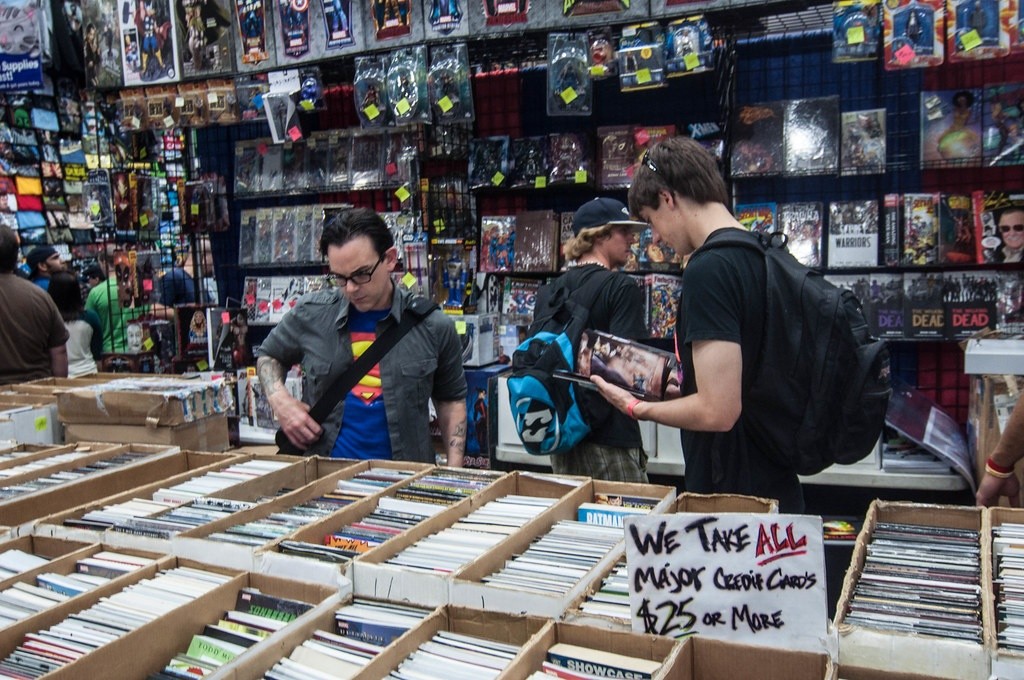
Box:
[987,205,1024,264]
[255,211,465,465]
[25,238,213,380]
[0,223,69,385]
[976,388,1024,511]
[589,137,806,515]
[525,196,649,486]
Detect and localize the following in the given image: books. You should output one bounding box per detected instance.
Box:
[726,85,1024,341]
[882,371,976,492]
[0,451,680,680]
[840,521,1024,652]
[553,329,679,401]
[0,94,229,279]
[477,124,725,366]
[234,127,421,431]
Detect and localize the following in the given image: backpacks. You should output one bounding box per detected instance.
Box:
[506,271,619,456]
[685,229,890,475]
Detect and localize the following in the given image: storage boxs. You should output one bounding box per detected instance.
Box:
[449,310,501,366]
[0,371,1024,680]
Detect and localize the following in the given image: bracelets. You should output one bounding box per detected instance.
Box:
[984,456,1015,478]
[627,399,642,421]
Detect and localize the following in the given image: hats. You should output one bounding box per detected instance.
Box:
[82,265,105,281]
[27,245,56,279]
[573,197,649,239]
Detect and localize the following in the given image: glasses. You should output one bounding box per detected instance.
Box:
[641,149,674,203]
[326,254,383,287]
[1000,224,1024,233]
